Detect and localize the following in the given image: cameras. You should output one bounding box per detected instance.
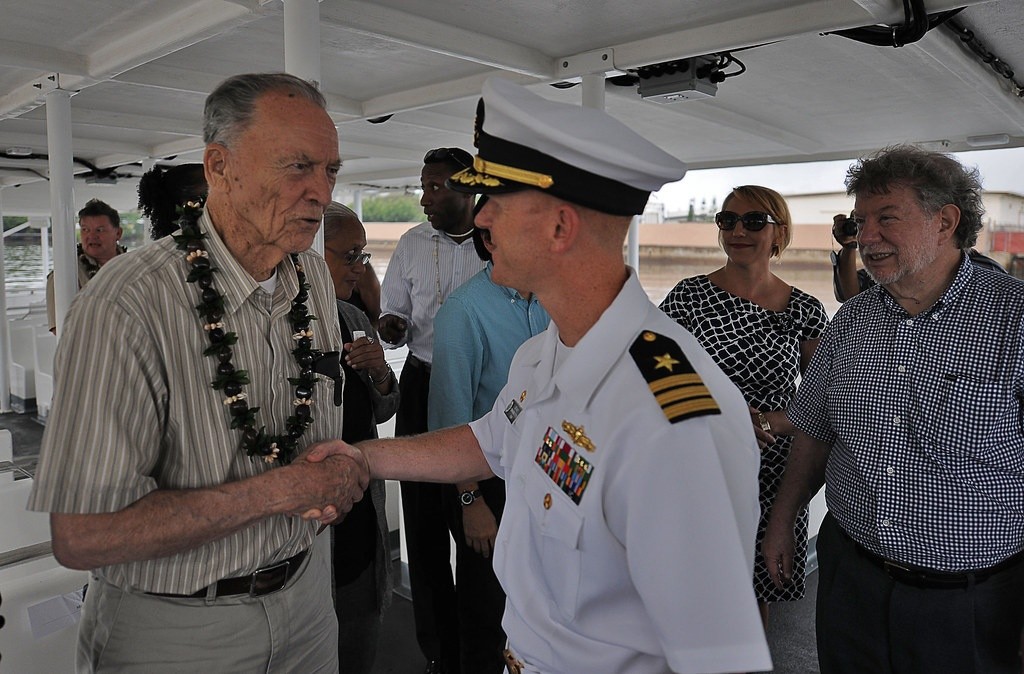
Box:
[841,218,858,236]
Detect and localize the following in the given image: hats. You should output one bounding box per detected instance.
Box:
[445,79,687,216]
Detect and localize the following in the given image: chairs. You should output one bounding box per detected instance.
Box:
[0,318,56,555]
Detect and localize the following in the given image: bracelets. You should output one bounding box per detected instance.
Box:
[368,363,391,385]
[756,411,771,433]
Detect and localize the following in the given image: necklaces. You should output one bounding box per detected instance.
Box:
[434,236,443,305]
[77,242,127,278]
[170,190,318,463]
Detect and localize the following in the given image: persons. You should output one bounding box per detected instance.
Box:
[27,72,1024,674]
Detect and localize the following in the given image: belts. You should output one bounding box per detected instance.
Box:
[407,355,432,373]
[144,549,311,599]
[844,536,1020,591]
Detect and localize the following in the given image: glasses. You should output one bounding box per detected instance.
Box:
[715,211,779,232]
[423,148,468,170]
[324,245,371,266]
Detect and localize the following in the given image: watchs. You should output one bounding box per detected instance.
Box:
[457,489,482,506]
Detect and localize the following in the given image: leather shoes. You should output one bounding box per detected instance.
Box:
[425,657,443,674]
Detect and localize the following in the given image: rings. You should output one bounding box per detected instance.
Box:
[367,336,375,344]
